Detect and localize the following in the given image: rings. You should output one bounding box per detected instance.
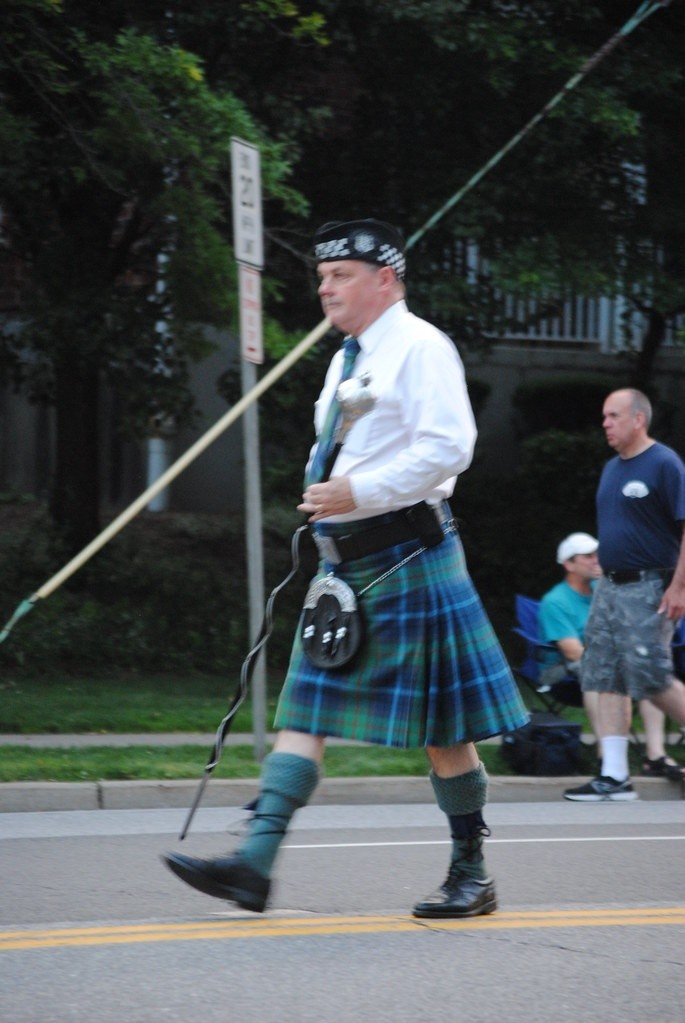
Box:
[315,504,323,512]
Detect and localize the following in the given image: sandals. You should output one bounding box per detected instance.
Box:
[641,755,685,778]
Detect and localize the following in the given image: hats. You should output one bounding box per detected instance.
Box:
[315,218,407,279]
[556,530,600,563]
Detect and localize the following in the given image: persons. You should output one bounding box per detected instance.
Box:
[535,531,685,782]
[158,219,532,919]
[562,388,685,802]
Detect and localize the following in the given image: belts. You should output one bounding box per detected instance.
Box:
[603,570,670,585]
[313,513,420,564]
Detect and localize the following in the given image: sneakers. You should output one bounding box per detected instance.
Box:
[564,774,638,800]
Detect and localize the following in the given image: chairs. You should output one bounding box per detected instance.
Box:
[497,594,648,768]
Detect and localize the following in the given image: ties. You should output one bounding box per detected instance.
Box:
[307,337,361,483]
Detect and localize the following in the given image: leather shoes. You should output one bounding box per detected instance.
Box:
[166,849,272,912]
[412,872,496,917]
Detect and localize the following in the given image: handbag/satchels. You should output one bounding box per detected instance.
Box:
[508,710,583,774]
[298,572,364,668]
